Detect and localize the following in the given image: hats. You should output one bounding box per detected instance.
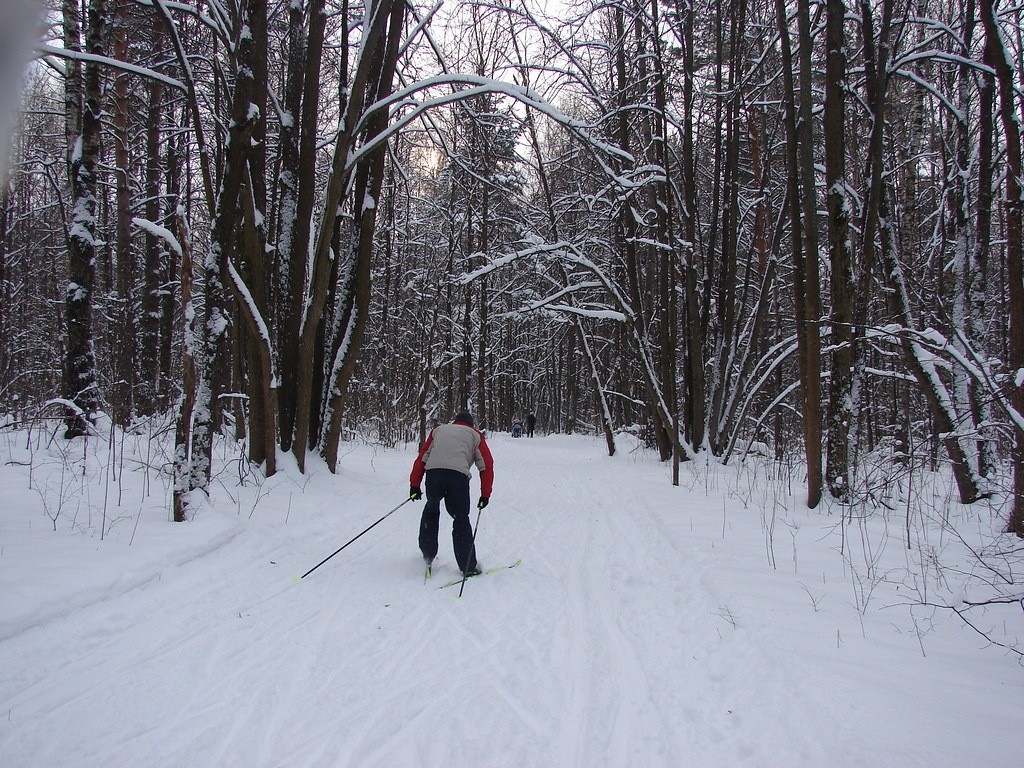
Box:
[456,411,474,426]
[530,412,534,415]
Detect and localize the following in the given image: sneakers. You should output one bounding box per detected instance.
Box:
[461,568,482,580]
[424,557,435,565]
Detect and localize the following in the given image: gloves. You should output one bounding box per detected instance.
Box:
[477,497,488,510]
[410,487,421,502]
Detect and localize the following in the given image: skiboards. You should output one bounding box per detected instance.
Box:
[422,542,522,600]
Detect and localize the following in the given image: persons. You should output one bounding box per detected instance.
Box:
[526,412,535,438]
[410,411,494,577]
[511,417,522,438]
